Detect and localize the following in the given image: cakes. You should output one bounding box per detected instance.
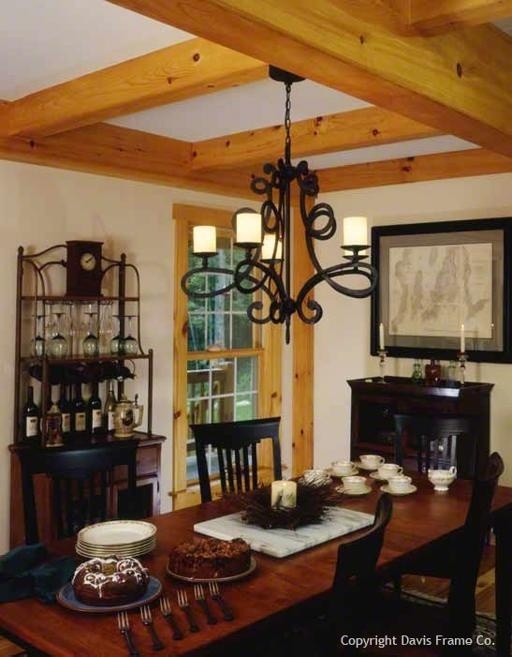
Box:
[169,536,251,577]
[69,557,149,607]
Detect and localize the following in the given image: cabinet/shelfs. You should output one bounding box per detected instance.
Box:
[14,243,153,450]
[346,376,496,480]
[7,431,167,550]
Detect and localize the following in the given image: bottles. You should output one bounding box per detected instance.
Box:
[103,381,117,434]
[39,386,54,432]
[85,383,102,433]
[57,385,71,435]
[22,386,39,439]
[71,384,85,432]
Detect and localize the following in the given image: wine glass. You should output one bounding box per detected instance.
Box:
[82,311,98,357]
[29,314,46,357]
[122,314,140,355]
[109,313,121,354]
[49,311,68,359]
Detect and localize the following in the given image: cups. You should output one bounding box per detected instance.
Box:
[360,454,385,467]
[387,476,412,491]
[377,463,403,475]
[331,460,357,475]
[303,469,331,483]
[341,476,367,491]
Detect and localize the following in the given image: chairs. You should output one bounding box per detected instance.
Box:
[250,493,392,654]
[389,450,505,639]
[15,435,144,547]
[188,416,285,505]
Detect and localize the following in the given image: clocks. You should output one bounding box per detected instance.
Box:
[65,238,105,299]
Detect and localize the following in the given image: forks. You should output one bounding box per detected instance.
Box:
[159,599,185,640]
[176,587,201,633]
[115,612,138,657]
[209,580,234,622]
[192,583,217,624]
[138,606,164,650]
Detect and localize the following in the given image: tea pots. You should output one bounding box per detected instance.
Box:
[111,398,144,437]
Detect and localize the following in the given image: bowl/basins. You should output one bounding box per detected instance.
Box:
[428,466,457,491]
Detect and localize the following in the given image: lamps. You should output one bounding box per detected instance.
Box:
[180,66,378,345]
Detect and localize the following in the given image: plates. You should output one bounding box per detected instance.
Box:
[167,556,258,583]
[326,468,360,477]
[356,462,380,471]
[74,520,157,560]
[379,485,417,496]
[298,478,333,488]
[369,472,404,481]
[335,485,372,496]
[56,576,162,614]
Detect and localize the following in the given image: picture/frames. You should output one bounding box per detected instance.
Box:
[368,216,512,364]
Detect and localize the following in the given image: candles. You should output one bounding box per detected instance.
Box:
[271,470,296,509]
[379,322,385,349]
[460,323,465,353]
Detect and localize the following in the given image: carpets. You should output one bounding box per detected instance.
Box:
[188,586,497,655]
